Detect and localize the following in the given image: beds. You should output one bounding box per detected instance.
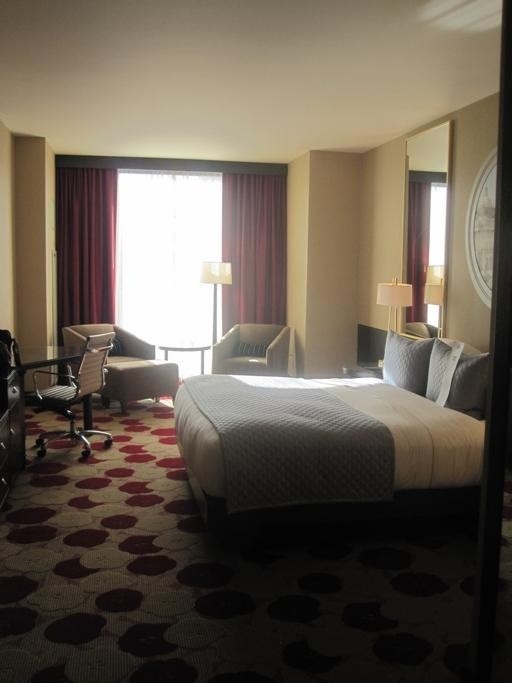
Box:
[173,326,492,534]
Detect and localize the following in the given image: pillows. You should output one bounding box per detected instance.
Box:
[236,342,269,357]
[404,320,437,337]
[382,327,438,398]
[426,338,491,421]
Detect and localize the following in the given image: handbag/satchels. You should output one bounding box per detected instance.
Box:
[0,330,11,377]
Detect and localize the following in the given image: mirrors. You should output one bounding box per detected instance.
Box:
[400,117,455,343]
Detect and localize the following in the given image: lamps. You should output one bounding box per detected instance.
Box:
[376,275,413,334]
[201,261,233,346]
[423,263,445,328]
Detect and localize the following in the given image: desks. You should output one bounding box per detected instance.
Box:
[15,342,95,438]
[156,341,212,375]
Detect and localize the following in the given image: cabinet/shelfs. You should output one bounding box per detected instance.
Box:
[1,364,28,510]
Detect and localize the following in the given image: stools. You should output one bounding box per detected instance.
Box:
[105,360,181,414]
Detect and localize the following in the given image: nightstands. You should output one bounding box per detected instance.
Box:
[342,365,384,380]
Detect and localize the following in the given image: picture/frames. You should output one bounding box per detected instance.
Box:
[462,142,498,309]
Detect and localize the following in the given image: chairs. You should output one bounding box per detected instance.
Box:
[62,321,156,408]
[212,322,291,376]
[27,330,117,459]
